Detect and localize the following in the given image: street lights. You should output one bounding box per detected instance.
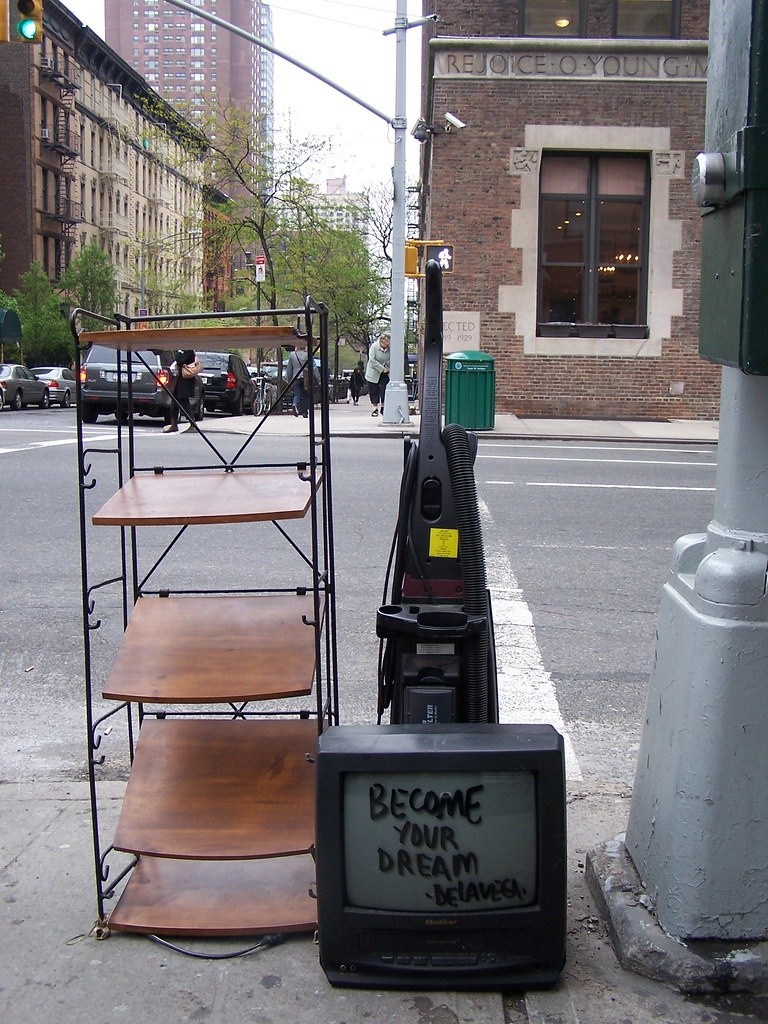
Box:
[140,229,202,308]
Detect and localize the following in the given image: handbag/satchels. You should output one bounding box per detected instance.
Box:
[303,370,310,392]
[180,349,202,379]
[354,385,360,393]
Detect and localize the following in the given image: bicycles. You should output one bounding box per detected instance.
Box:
[249,375,273,417]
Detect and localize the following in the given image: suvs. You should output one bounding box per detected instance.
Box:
[79,343,206,425]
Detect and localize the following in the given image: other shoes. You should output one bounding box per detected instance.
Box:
[372,409,378,418]
[292,405,298,417]
[164,427,178,433]
[182,425,197,433]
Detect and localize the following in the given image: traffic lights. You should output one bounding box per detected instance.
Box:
[16,0,43,43]
[424,244,456,275]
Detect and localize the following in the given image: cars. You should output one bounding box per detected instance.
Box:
[243,358,355,414]
[0,363,51,411]
[194,351,259,416]
[30,367,76,408]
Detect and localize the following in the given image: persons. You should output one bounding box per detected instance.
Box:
[287,345,321,418]
[163,350,198,433]
[365,333,391,417]
[349,368,364,406]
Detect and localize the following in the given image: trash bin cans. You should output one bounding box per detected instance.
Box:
[443,351,496,431]
